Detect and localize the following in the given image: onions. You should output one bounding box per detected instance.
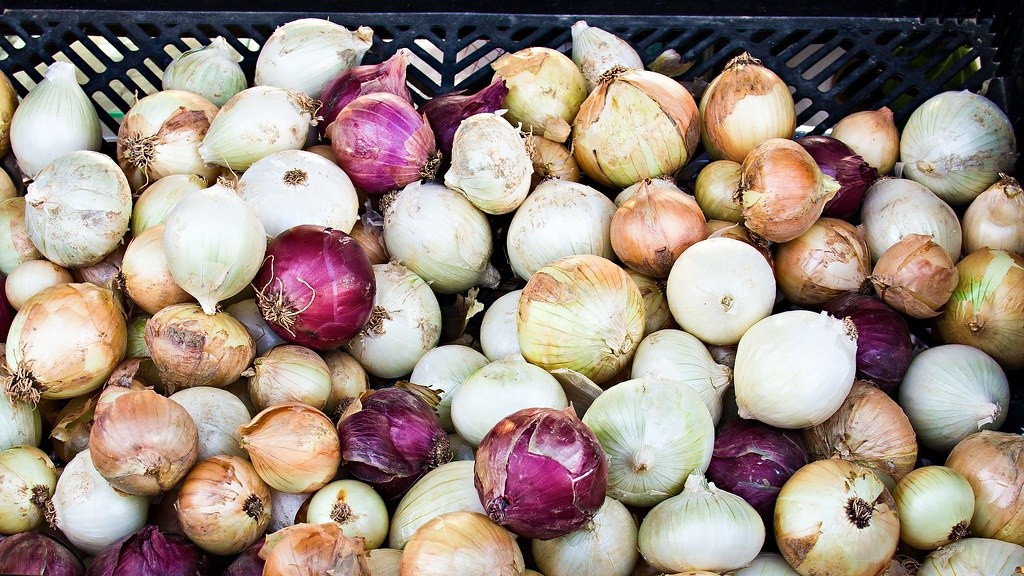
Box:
[0,18,1024,576]
